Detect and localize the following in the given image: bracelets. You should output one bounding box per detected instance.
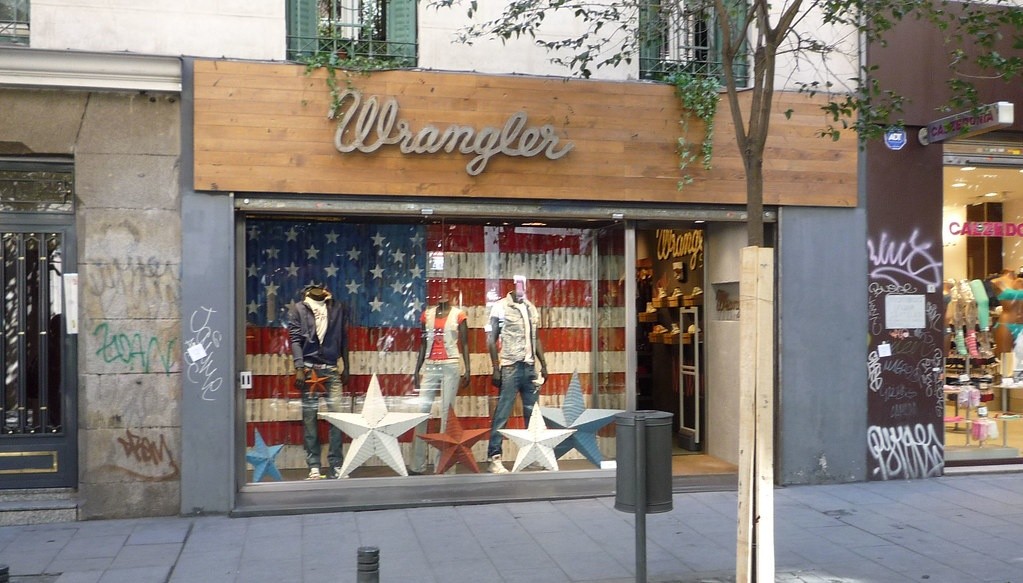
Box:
[541,360,544,363]
[492,359,499,367]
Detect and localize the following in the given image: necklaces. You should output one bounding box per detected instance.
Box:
[438,308,450,314]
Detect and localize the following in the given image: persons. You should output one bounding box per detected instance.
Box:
[288,287,349,481]
[406,299,470,476]
[969,279,993,357]
[990,269,1023,385]
[959,279,981,358]
[944,280,952,358]
[487,275,549,474]
[949,278,967,356]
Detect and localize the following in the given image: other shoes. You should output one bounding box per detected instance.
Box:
[308,469,321,480]
[330,466,350,479]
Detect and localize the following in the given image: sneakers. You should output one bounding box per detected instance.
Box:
[672,288,683,297]
[486,454,509,473]
[670,324,680,335]
[687,324,702,334]
[646,303,657,313]
[692,286,703,296]
[658,287,667,299]
[653,325,668,334]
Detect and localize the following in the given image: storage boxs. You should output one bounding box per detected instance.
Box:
[683,333,703,344]
[649,333,663,343]
[663,334,680,344]
[668,297,680,307]
[683,295,703,306]
[639,313,657,322]
[652,298,666,307]
